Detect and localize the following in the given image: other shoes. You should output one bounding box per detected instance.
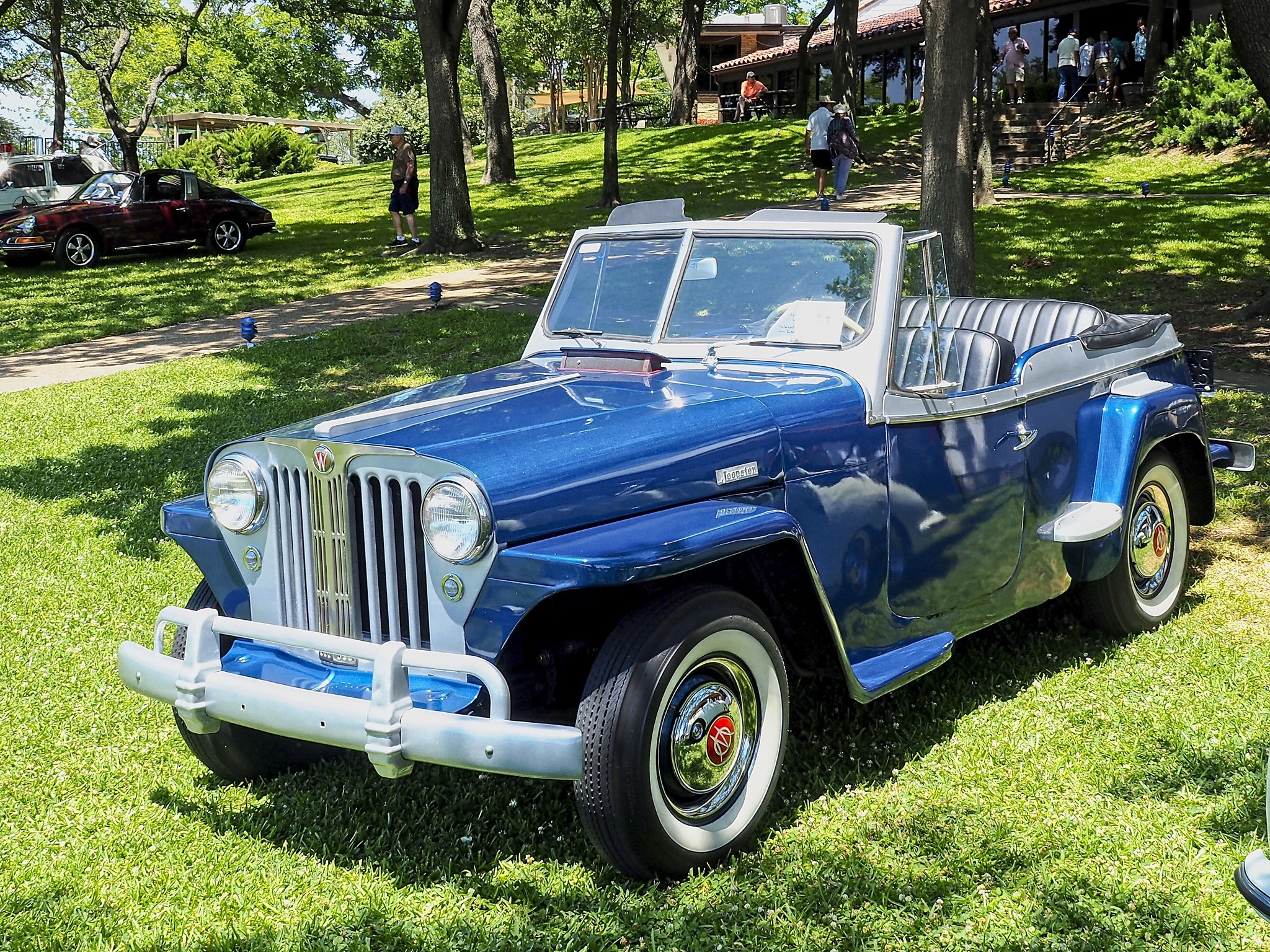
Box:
[1017,97,1023,104]
[816,194,826,200]
[836,194,846,200]
[739,112,745,118]
[1007,101,1014,105]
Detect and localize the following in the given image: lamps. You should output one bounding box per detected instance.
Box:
[1141,182,1150,195]
[1004,162,1012,173]
[822,198,831,211]
[1047,128,1055,137]
[1100,79,1106,87]
[427,282,444,302]
[240,316,259,340]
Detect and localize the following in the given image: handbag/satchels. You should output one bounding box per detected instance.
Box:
[832,128,857,161]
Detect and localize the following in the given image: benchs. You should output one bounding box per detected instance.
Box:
[719,104,795,111]
[720,90,794,115]
[839,295,1107,360]
[587,115,624,122]
[625,116,659,120]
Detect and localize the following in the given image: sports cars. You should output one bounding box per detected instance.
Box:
[0,169,279,268]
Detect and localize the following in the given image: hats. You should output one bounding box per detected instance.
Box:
[919,40,926,47]
[384,125,404,138]
[746,72,756,79]
[819,95,837,103]
[831,104,850,118]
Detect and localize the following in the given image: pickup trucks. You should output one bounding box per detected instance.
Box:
[120,195,1257,878]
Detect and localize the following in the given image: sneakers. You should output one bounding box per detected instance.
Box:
[386,238,408,247]
[407,239,422,246]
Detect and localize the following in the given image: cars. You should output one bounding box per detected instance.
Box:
[0,142,138,213]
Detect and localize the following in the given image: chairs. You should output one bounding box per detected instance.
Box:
[159,185,175,200]
[891,327,1014,395]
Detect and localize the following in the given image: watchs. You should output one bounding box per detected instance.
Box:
[744,98,747,100]
[403,180,408,185]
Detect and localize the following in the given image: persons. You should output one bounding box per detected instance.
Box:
[973,26,1030,105]
[50,135,122,171]
[385,126,422,247]
[910,41,926,114]
[805,96,860,202]
[731,72,769,123]
[1056,17,1146,101]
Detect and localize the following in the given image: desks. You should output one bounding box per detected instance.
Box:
[598,102,655,131]
